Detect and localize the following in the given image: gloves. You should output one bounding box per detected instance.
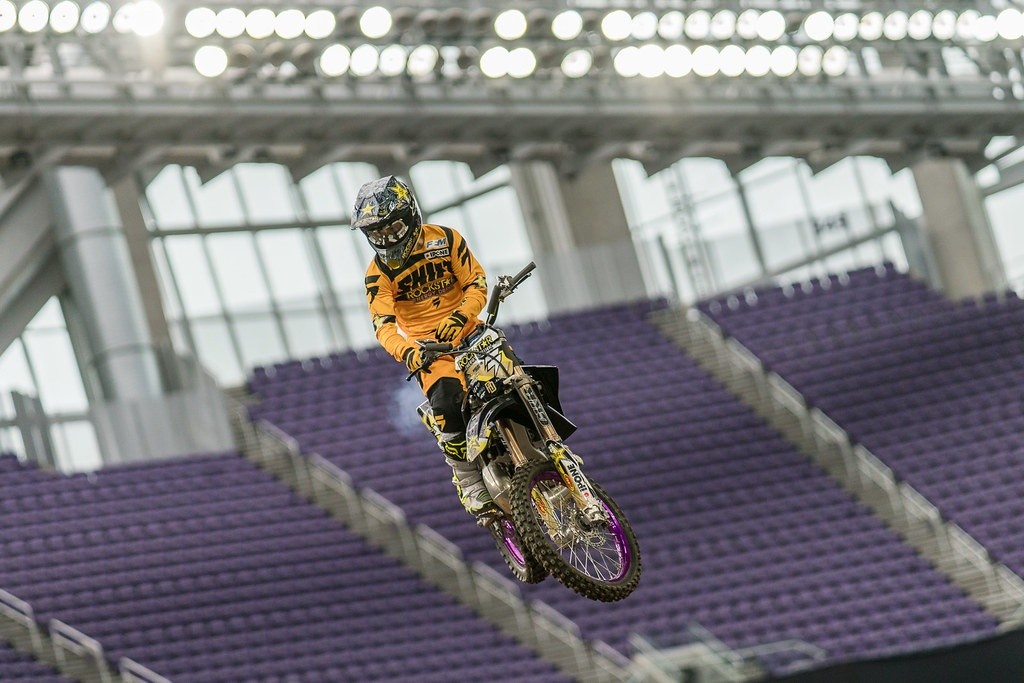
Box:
[435,311,467,343]
[402,347,432,374]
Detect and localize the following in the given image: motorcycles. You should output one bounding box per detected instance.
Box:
[406,262,643,604]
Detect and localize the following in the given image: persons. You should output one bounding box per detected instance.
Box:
[350,174,585,518]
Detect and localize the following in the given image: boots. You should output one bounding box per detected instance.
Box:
[437,432,503,519]
[563,445,584,465]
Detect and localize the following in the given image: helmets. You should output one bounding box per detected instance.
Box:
[350,175,423,275]
